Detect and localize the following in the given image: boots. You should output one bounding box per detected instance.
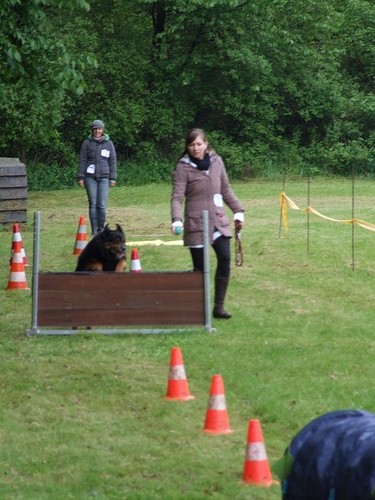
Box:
[90,217,105,235]
[213,277,232,318]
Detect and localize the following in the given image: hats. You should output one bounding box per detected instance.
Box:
[90,120,105,129]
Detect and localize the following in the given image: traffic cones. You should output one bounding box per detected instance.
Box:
[241,419,279,487]
[4,244,31,291]
[72,216,89,255]
[203,374,233,435]
[129,248,142,273]
[163,347,194,401]
[9,223,29,267]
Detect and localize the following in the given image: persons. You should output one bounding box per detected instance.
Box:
[77,119,117,235]
[172,129,246,319]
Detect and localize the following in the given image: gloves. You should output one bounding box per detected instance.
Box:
[233,212,244,222]
[171,220,183,235]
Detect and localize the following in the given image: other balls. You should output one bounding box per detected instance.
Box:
[175,226,183,235]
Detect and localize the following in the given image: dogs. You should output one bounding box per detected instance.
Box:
[71,222,127,273]
